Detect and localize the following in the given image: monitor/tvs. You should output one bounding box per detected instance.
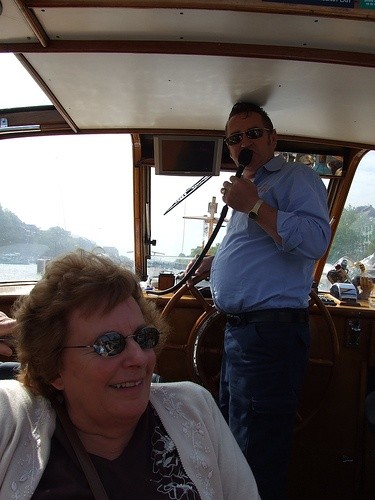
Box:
[154,135,223,176]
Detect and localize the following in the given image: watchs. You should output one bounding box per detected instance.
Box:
[248,199,264,220]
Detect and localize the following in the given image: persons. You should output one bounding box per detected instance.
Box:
[185,102,331,500]
[0,248,261,500]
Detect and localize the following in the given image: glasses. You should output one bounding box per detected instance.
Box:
[63,324,160,360]
[223,127,272,147]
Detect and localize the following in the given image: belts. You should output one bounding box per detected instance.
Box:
[222,308,309,327]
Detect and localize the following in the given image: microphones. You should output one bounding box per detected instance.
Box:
[235,149,253,179]
